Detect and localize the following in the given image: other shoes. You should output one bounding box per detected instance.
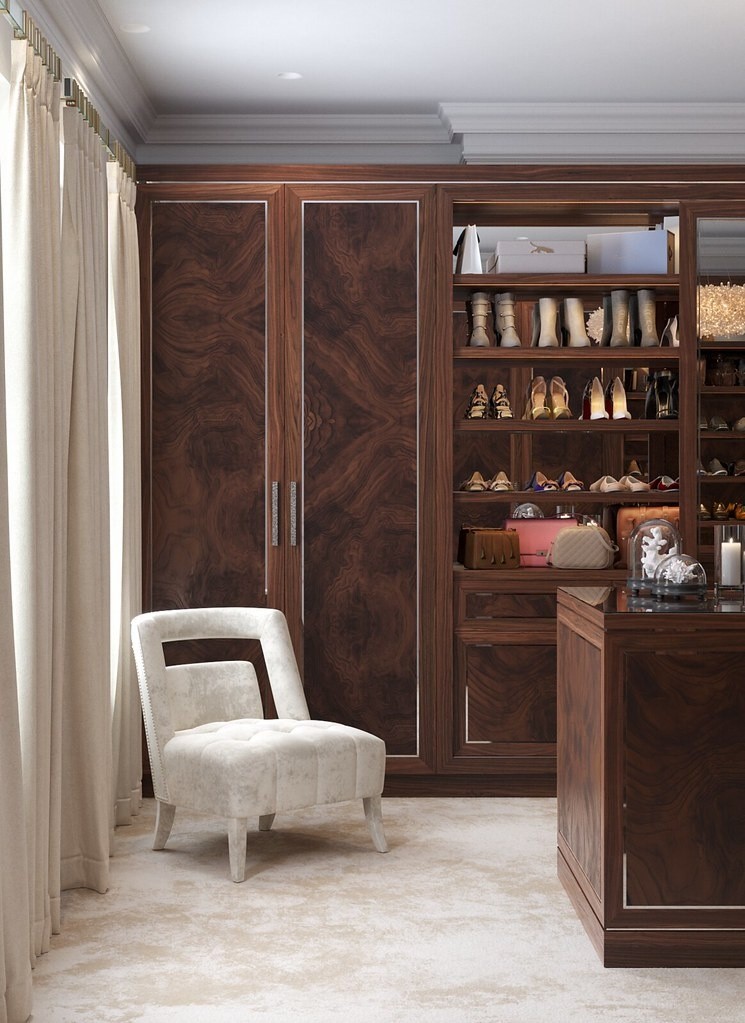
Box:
[732,417,745,431]
[626,461,640,474]
[735,504,745,520]
[532,472,583,492]
[700,417,728,430]
[699,501,729,521]
[600,475,651,492]
[466,472,513,491]
[659,476,678,492]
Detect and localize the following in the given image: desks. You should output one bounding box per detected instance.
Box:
[553,582,743,972]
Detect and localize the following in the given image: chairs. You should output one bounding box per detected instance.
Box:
[131,605,389,885]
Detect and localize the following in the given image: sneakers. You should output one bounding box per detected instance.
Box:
[697,459,727,475]
[734,459,745,476]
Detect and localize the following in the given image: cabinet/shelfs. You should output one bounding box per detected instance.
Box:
[688,162,745,801]
[443,163,688,796]
[132,162,438,800]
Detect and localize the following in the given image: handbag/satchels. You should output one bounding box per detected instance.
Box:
[503,518,578,567]
[618,506,683,569]
[454,224,485,274]
[465,530,519,569]
[546,525,619,569]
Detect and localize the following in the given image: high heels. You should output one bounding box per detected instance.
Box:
[532,375,572,420]
[660,315,680,347]
[468,384,512,419]
[591,377,632,420]
[654,370,679,419]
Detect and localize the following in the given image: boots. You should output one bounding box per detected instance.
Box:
[611,290,658,347]
[539,298,591,348]
[470,292,520,347]
